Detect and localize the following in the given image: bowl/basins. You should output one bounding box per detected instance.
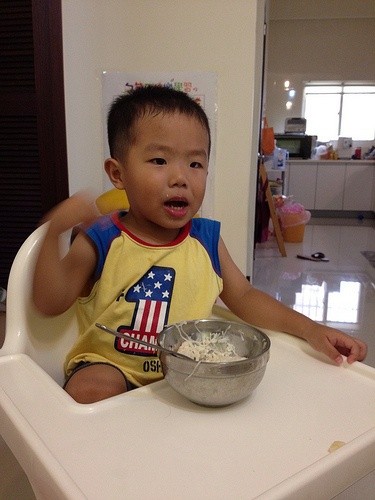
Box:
[156,318,271,407]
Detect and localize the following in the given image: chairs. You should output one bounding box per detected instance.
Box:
[0,218,375,500]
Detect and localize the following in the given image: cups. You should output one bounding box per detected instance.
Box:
[355,148,361,159]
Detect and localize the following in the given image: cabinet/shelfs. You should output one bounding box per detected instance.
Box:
[283,163,375,218]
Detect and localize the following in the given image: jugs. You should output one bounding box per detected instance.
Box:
[274,148,289,169]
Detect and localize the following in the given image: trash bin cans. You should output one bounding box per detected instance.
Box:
[279,203,306,242]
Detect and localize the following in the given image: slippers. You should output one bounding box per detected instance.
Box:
[297,252,329,262]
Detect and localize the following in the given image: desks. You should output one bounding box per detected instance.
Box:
[285,160,375,163]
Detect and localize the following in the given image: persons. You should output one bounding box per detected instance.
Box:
[33,81,367,405]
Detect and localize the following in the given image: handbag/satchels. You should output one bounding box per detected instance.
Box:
[261,118,274,155]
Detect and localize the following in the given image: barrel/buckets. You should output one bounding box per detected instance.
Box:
[280,212,305,243]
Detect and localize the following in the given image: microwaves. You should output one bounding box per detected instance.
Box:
[274,134,317,160]
